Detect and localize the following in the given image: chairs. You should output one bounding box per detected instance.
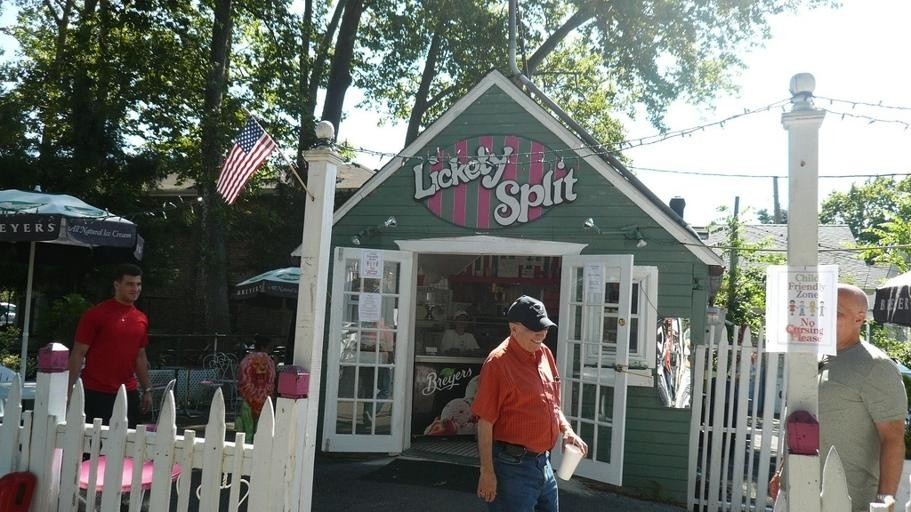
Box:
[194,351,244,418]
[145,379,177,432]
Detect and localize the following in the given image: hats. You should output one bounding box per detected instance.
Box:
[507,295,559,330]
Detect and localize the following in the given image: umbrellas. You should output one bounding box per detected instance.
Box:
[0,179,137,382]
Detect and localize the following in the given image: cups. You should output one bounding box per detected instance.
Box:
[555,444,585,480]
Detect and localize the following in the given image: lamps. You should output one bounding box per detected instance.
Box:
[350,215,398,246]
[582,218,647,247]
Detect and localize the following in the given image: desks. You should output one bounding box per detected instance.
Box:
[80,455,182,512]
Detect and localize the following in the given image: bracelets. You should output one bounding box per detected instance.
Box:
[143,386,154,392]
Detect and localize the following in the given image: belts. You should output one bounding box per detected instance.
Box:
[494,440,545,458]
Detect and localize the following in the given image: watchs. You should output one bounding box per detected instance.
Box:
[875,493,896,505]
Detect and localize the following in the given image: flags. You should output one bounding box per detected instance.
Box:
[215,112,277,207]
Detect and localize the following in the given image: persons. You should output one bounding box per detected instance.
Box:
[474,294,589,512]
[768,281,909,511]
[63,262,156,464]
